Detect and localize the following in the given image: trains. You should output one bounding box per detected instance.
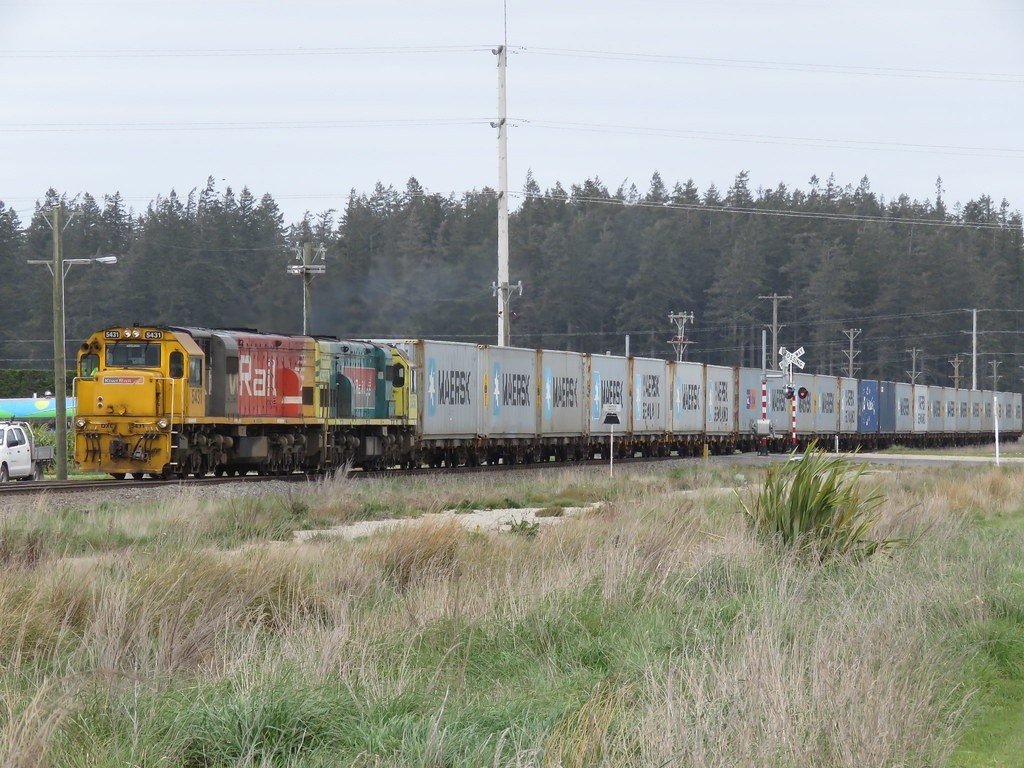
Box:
[71,324,1024,480]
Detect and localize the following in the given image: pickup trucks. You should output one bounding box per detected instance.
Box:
[1,419,54,485]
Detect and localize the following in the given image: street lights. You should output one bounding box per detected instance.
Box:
[27,203,117,480]
